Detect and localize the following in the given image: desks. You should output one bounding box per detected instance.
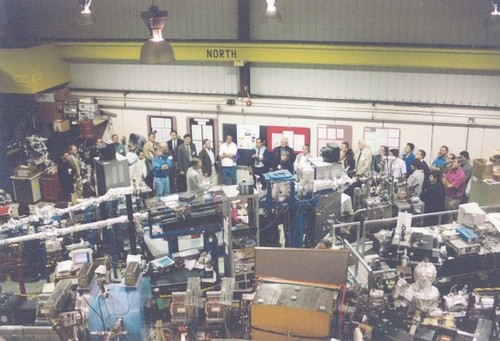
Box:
[11,169,41,204]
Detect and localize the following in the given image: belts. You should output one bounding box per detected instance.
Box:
[211,163,215,167]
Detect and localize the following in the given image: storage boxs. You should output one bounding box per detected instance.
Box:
[16,165,38,178]
[77,97,101,122]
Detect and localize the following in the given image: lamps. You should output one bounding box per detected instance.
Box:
[490,0,500,16]
[261,0,281,23]
[78,0,92,14]
[139,0,177,65]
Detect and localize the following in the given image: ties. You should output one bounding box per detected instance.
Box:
[357,150,361,162]
[173,140,175,148]
[256,148,261,165]
[186,144,191,156]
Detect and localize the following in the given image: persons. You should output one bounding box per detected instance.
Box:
[58,144,91,202]
[318,238,332,250]
[91,131,174,196]
[219,134,238,185]
[341,139,472,226]
[249,138,272,180]
[271,139,295,175]
[292,143,314,173]
[167,130,220,191]
